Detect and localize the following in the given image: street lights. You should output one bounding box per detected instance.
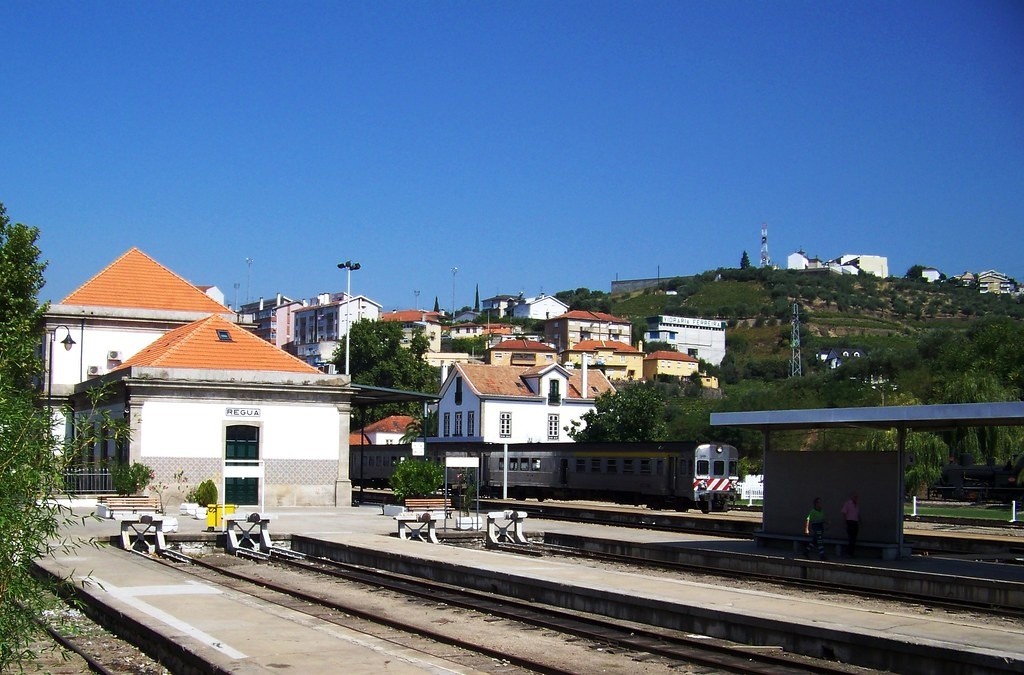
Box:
[451,267,458,324]
[46,324,76,456]
[234,282,240,313]
[414,291,420,311]
[337,260,362,376]
[245,256,253,305]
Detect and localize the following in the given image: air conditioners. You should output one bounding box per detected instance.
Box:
[107,350,122,361]
[323,364,336,375]
[87,365,104,376]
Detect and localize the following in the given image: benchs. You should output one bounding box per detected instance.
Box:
[95,495,165,519]
[404,499,456,519]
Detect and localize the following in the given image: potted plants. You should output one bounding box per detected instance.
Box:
[195,479,218,519]
[147,473,189,533]
[451,470,483,529]
[179,486,200,515]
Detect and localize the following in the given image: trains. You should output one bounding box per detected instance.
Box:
[933,452,1024,502]
[349,440,739,514]
